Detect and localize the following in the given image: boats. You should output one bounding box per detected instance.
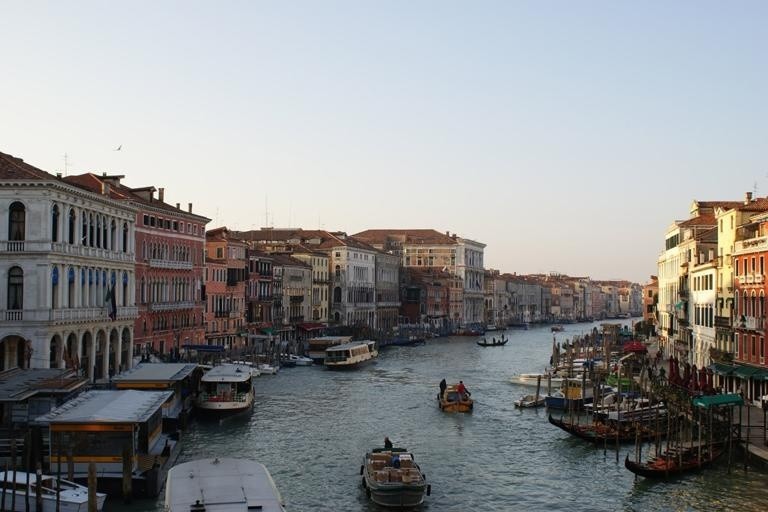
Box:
[112,361,199,427]
[436,378,473,412]
[180,322,381,371]
[163,456,283,511]
[360,437,432,507]
[197,363,255,416]
[476,334,508,346]
[1,470,107,512]
[1,367,91,462]
[29,389,184,498]
[507,325,735,477]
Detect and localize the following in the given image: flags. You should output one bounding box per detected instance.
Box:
[110,283,117,321]
[105,284,114,321]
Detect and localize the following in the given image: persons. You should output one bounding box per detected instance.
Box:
[438,377,447,400]
[456,380,465,403]
[391,452,400,470]
[382,435,391,450]
